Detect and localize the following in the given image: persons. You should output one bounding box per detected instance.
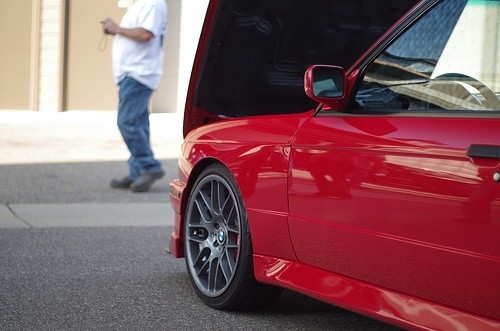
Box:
[100,0,167,192]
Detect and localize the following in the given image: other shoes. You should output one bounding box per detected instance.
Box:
[111,173,140,189]
[130,166,165,192]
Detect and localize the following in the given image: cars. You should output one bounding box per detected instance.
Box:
[167,1,500,331]
